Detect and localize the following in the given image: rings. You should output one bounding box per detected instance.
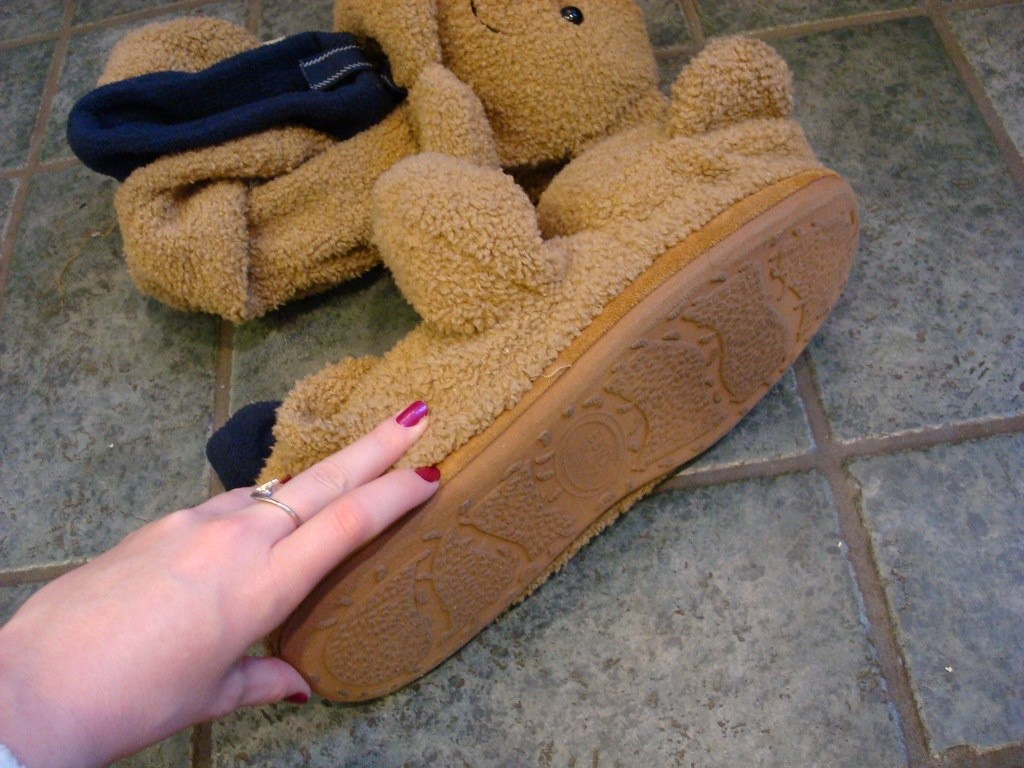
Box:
[250,479,302,527]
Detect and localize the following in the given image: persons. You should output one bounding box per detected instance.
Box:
[0,401,441,768]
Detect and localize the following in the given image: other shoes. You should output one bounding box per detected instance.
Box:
[69,1,667,326]
[208,41,857,703]
